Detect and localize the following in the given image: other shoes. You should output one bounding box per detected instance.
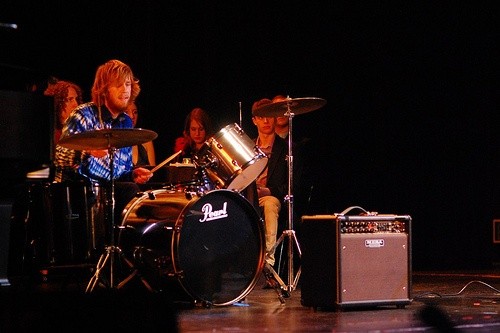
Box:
[264,278,279,288]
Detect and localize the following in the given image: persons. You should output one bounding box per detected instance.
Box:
[175,108,216,162]
[125,103,156,191]
[251,99,289,288]
[53,60,153,230]
[273,96,289,142]
[44,81,82,164]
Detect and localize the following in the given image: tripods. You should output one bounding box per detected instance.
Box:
[265,103,302,294]
[84,137,153,295]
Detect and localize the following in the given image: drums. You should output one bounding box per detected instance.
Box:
[192,123,268,192]
[113,187,266,306]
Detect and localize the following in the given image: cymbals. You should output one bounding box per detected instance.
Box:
[59,128,158,150]
[252,97,328,116]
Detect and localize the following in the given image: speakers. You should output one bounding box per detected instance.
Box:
[300,214,413,310]
[0,90,55,164]
